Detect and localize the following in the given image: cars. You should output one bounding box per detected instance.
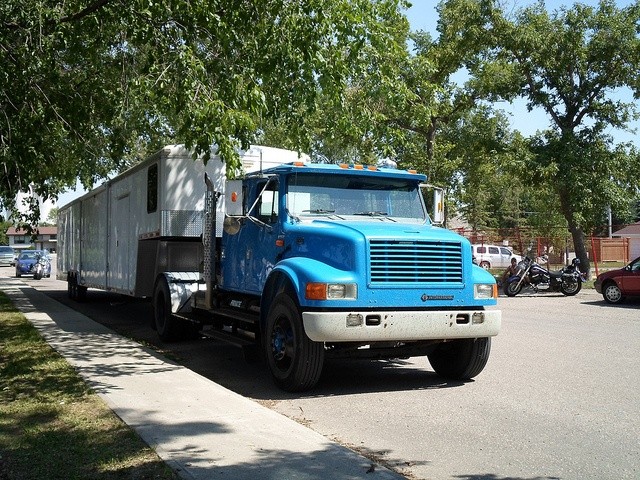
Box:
[16,249,53,278]
[0,246,15,267]
[594,256,640,304]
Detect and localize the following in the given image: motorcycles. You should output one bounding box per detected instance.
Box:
[504,255,587,296]
[33,253,46,279]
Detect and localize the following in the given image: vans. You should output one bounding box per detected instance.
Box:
[472,244,525,269]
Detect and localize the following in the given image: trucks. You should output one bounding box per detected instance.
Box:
[56,143,499,391]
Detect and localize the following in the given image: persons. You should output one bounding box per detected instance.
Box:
[498,257,530,290]
